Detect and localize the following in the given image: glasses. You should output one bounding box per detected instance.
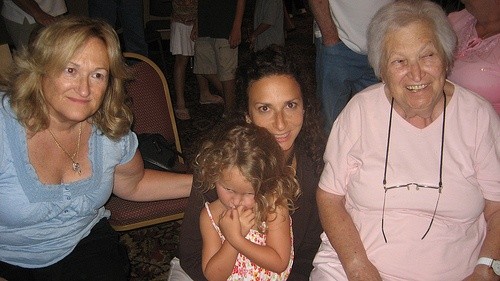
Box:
[382,183,442,243]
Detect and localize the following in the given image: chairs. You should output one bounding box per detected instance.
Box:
[102,53,190,232]
[118,29,162,51]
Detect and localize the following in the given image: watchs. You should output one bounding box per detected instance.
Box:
[474,255,500,275]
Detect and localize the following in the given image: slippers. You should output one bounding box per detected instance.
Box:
[174,100,191,120]
[199,94,225,105]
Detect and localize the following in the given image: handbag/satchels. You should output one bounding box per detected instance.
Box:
[138,133,188,174]
[284,4,296,31]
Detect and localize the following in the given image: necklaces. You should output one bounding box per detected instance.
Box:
[44,121,83,177]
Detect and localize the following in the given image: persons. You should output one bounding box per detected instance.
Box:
[168,43,330,281]
[447,0,500,114]
[0,16,193,281]
[310,0,500,281]
[189,124,304,281]
[1,0,73,55]
[309,0,400,140]
[88,0,295,121]
